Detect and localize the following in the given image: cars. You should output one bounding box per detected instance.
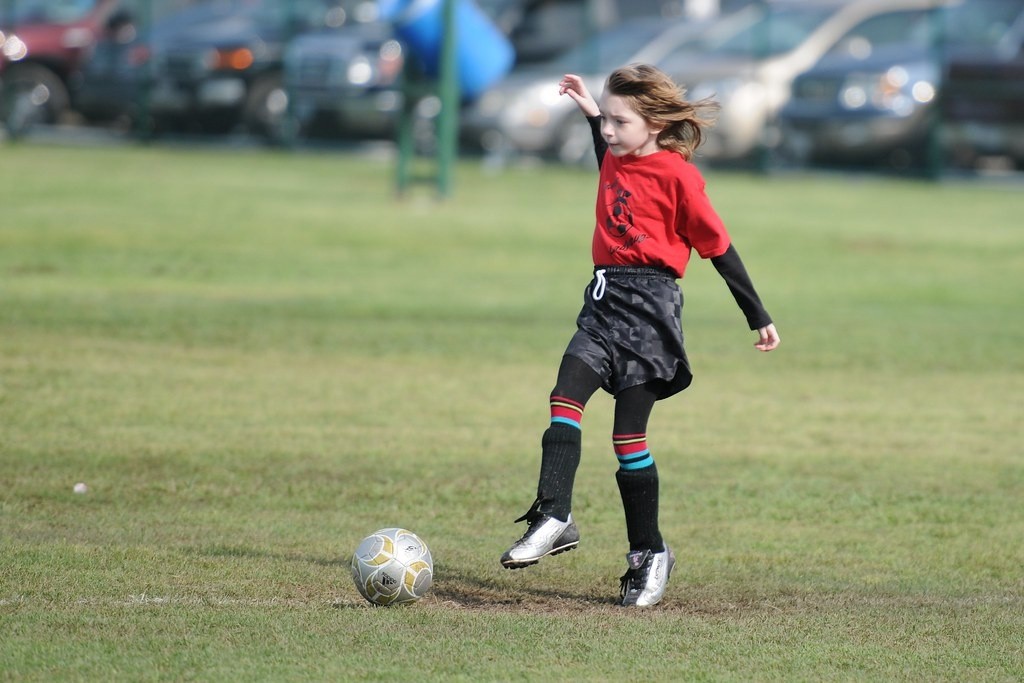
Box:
[648,3,952,171]
[0,0,410,146]
[779,10,1023,176]
[469,13,705,166]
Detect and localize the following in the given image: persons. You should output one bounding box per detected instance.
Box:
[500,62,782,606]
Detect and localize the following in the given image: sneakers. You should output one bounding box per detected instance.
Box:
[622,542,676,608]
[501,512,578,569]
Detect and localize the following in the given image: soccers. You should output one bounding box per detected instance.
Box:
[350,528,435,609]
